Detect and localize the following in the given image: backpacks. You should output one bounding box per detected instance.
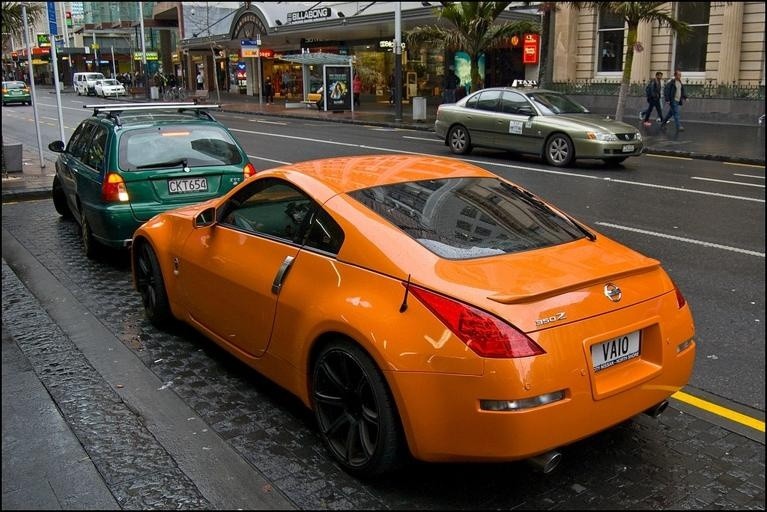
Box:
[646,79,656,96]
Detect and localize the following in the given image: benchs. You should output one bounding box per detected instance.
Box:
[300,91,323,108]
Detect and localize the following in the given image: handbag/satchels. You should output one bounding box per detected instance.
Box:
[679,96,686,105]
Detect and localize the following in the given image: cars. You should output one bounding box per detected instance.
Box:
[430,83,646,170]
[1,80,32,106]
[93,77,126,99]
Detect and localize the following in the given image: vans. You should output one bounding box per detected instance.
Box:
[72,72,106,96]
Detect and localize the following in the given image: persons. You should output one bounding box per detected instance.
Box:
[115,71,177,96]
[638,71,671,127]
[330,82,344,101]
[488,64,505,88]
[352,74,364,108]
[444,69,460,103]
[440,70,450,103]
[196,71,205,90]
[2,70,64,88]
[264,74,275,105]
[387,68,396,106]
[658,69,687,131]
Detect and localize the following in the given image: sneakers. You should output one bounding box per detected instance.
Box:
[639,111,685,131]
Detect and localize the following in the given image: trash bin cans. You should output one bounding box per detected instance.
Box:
[413,97,427,120]
[59,81,64,91]
[151,86,159,99]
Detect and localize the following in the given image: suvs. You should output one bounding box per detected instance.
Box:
[48,99,257,263]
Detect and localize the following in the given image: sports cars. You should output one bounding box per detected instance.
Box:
[129,149,700,485]
